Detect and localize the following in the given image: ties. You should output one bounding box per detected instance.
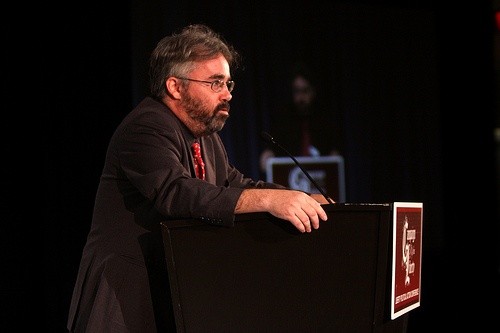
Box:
[191,138,206,183]
[302,121,310,155]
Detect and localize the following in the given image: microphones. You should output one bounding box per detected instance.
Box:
[261,133,336,204]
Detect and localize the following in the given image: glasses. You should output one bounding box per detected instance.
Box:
[164,76,235,93]
[292,86,312,95]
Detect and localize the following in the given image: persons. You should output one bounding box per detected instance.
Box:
[259,71,339,175]
[66,25,336,333]
